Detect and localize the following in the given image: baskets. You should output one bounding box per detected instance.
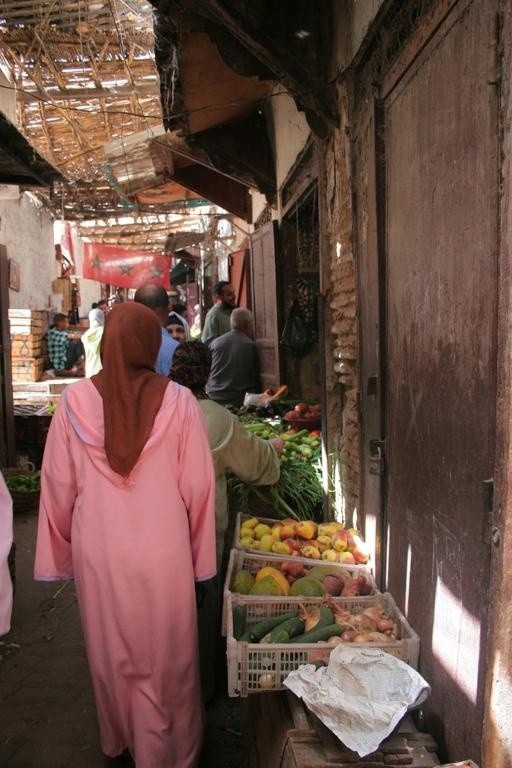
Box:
[221,512,420,697]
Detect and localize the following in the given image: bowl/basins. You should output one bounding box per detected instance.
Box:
[283,417,322,430]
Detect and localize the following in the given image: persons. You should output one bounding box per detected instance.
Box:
[47,301,109,379]
[110,287,124,304]
[170,341,283,705]
[34,303,217,768]
[136,282,259,406]
[1,476,14,636]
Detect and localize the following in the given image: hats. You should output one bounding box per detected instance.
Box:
[168,315,182,324]
